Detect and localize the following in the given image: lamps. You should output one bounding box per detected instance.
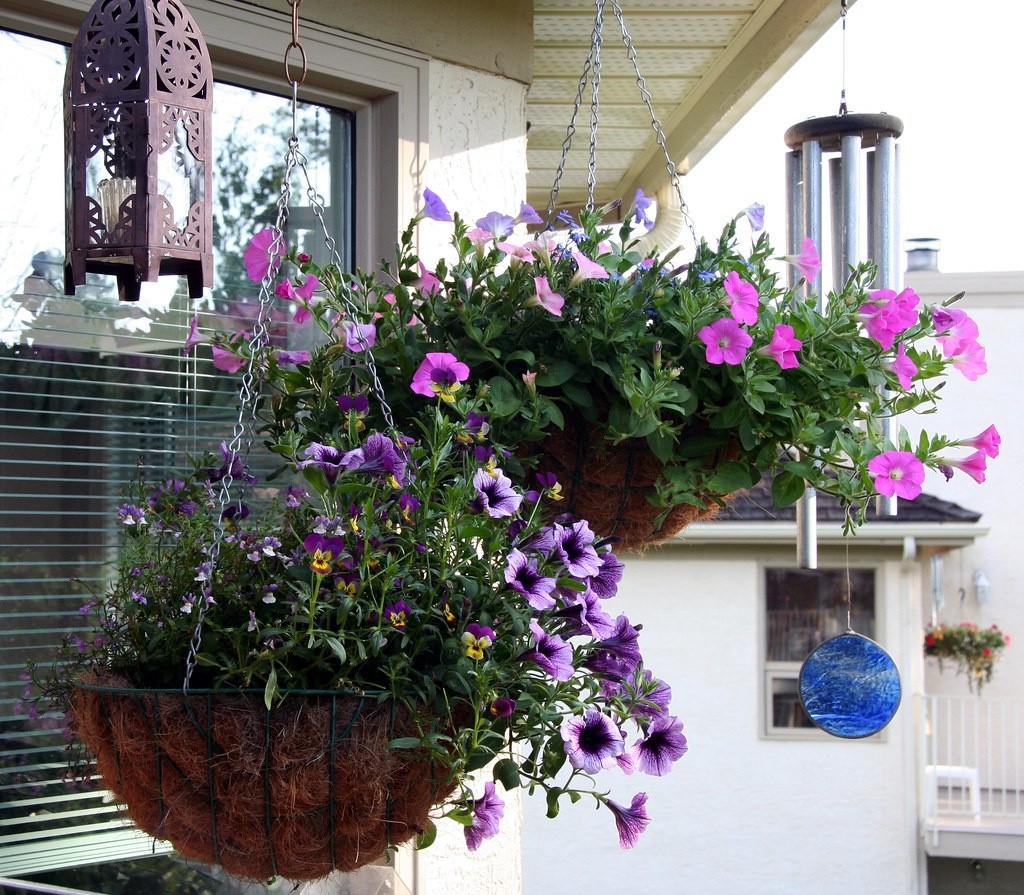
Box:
[61,0,214,300]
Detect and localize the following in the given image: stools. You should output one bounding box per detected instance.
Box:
[925,766,981,824]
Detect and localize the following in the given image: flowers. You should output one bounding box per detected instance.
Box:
[40,194,1005,850]
